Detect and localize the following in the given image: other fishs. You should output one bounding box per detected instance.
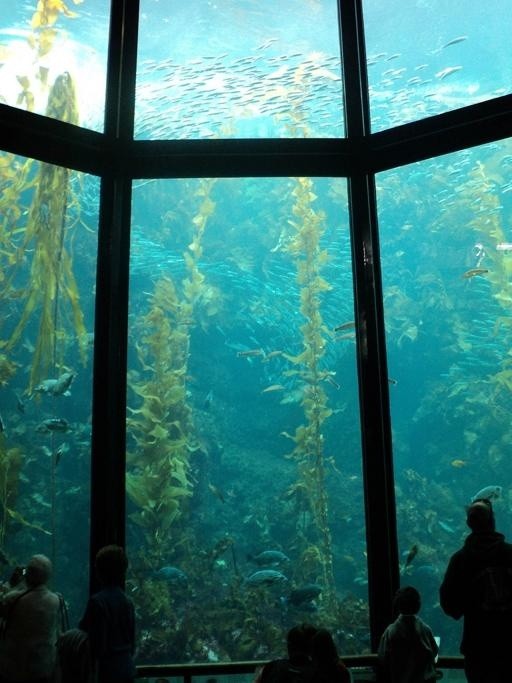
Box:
[69,29,512,394]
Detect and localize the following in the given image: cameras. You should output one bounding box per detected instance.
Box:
[20,566,28,575]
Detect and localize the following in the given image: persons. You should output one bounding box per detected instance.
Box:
[306,626,352,681]
[373,584,445,682]
[0,555,69,682]
[79,545,135,683]
[254,622,316,682]
[439,498,511,682]
[50,628,101,682]
